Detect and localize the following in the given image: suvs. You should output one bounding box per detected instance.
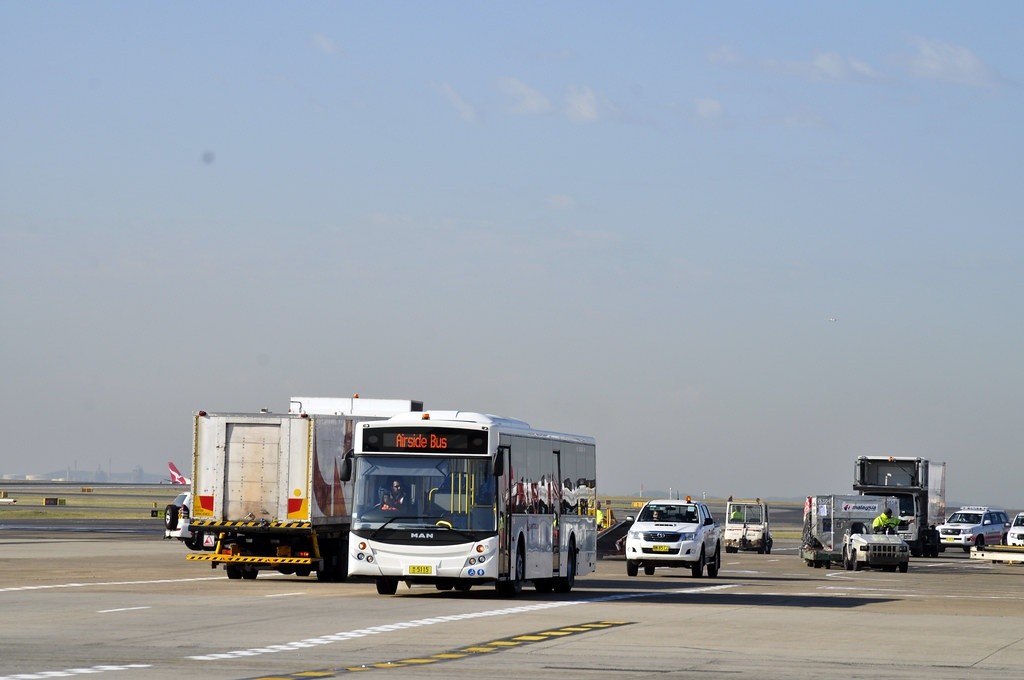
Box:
[163,491,203,550]
[936,505,1012,552]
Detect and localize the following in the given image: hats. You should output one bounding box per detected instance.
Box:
[884,508,892,515]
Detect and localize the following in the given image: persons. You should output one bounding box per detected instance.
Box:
[872,508,913,534]
[730,506,744,521]
[597,502,604,529]
[381,477,409,510]
[653,510,663,520]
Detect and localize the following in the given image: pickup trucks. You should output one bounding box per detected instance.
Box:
[626,496,721,577]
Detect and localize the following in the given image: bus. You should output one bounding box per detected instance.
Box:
[338,409,597,597]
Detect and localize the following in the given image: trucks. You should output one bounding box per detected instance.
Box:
[798,494,910,575]
[724,495,773,554]
[853,455,946,556]
[186,410,392,583]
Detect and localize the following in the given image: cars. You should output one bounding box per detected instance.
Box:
[1004,512,1024,546]
